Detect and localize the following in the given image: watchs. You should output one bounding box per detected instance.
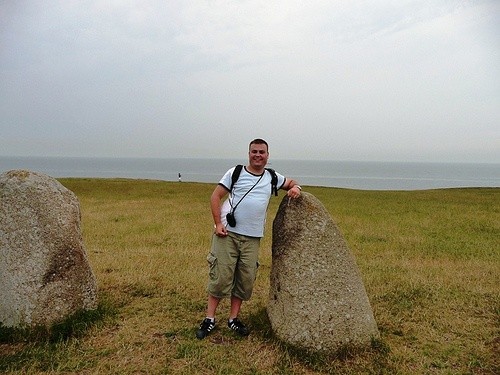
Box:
[297,186,301,191]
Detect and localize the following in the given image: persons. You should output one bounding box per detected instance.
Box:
[195,139,302,338]
[179,172,182,182]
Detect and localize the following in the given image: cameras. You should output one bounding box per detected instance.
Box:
[226,213,236,227]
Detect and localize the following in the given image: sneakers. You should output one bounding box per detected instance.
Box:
[228,315,251,338]
[197,316,216,340]
